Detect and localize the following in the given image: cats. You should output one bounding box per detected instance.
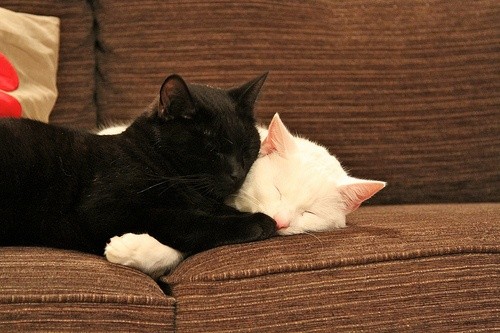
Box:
[93,110,388,283]
[0,69,281,251]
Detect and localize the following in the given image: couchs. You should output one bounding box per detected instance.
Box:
[0,0,499,332]
